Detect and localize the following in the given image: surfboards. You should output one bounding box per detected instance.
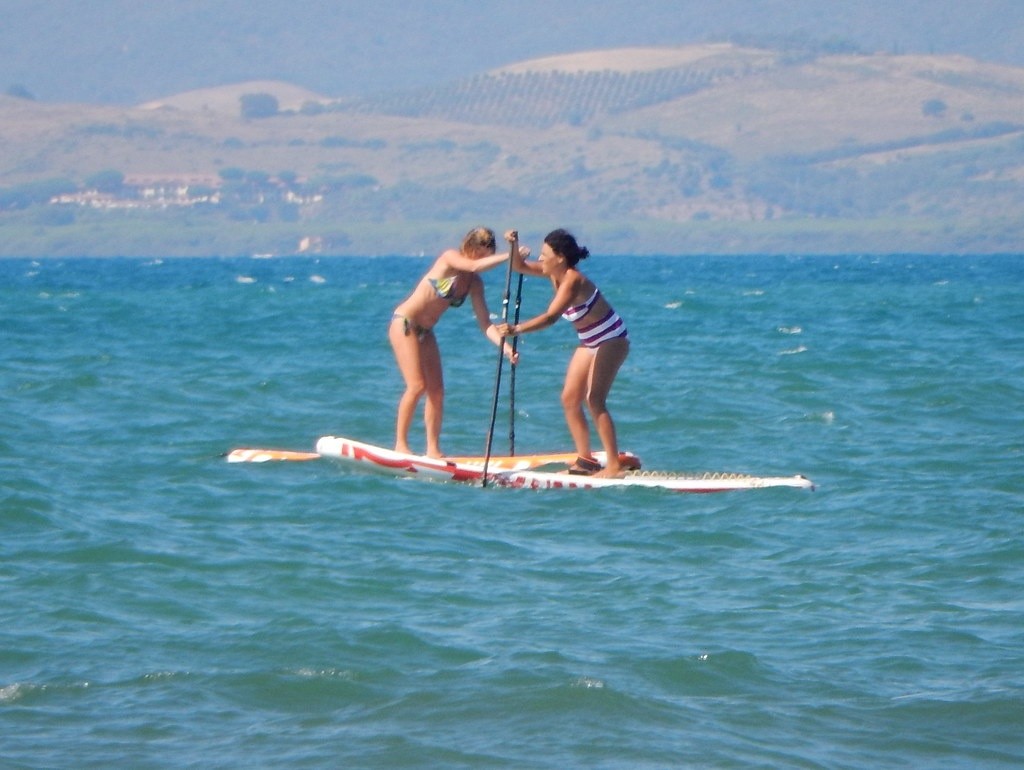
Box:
[315,435,815,491]
[229,446,642,471]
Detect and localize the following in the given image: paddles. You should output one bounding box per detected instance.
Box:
[508,275,523,457]
[480,230,519,487]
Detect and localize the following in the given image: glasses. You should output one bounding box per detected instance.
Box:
[480,241,492,248]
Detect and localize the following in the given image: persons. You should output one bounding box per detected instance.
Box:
[389,226,532,457]
[504,228,630,478]
